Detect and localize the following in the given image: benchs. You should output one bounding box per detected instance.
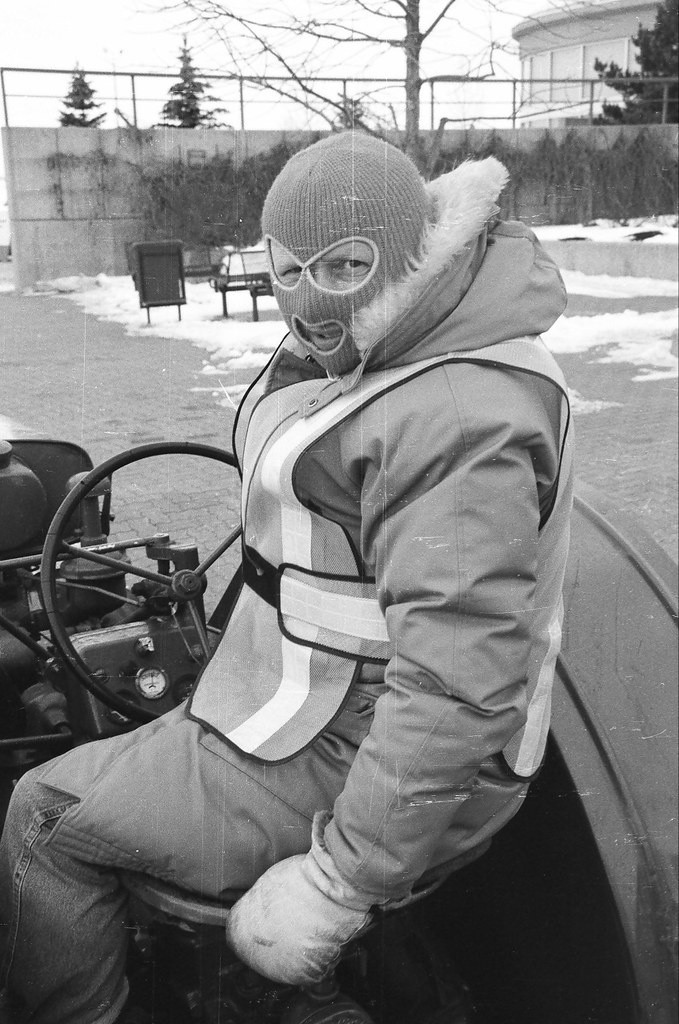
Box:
[210,245,274,322]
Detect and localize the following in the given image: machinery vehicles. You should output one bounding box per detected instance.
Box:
[0,437,679,1023]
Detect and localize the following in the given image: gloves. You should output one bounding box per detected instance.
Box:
[226,811,376,988]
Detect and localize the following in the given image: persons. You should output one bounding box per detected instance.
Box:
[0,129,574,1024]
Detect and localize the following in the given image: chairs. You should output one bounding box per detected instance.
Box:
[69,624,545,1023]
